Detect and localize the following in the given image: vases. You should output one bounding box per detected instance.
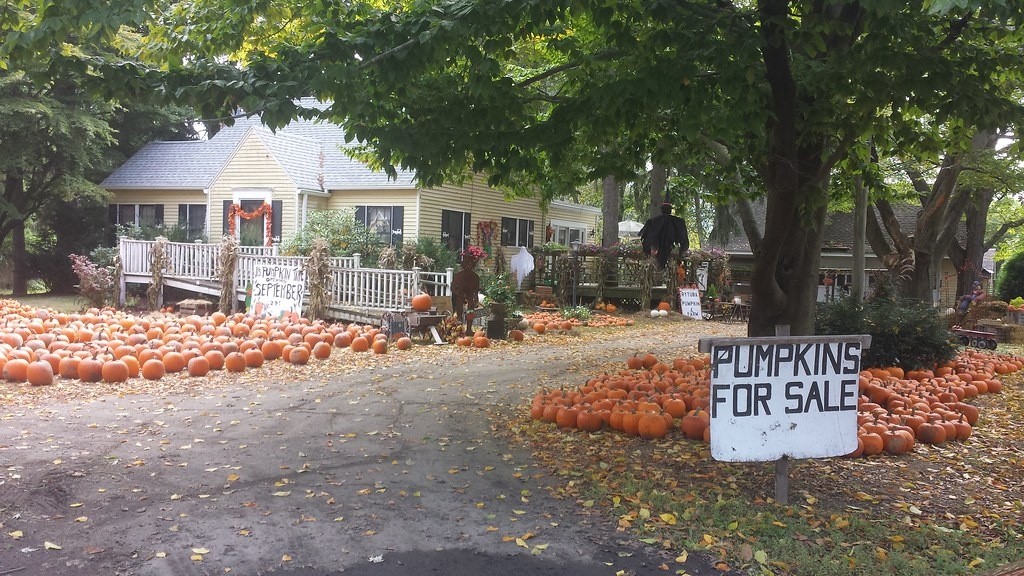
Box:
[489,302,507,321]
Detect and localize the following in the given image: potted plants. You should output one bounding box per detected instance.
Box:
[705,284,718,302]
[505,312,524,330]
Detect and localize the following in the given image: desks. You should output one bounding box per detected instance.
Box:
[702,302,726,321]
[728,303,747,323]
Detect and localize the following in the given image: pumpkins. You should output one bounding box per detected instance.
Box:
[531,349,1024,458]
[0,297,413,386]
[1007,304,1024,311]
[412,292,432,312]
[457,301,669,348]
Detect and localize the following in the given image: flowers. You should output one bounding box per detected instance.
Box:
[478,269,516,321]
[460,244,488,271]
